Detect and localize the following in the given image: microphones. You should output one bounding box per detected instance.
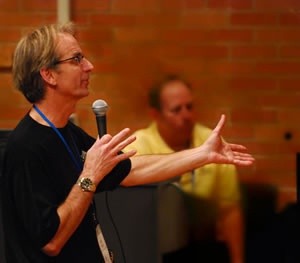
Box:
[92,99,108,139]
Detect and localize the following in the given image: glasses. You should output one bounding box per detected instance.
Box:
[51,54,84,66]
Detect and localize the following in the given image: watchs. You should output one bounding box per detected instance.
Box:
[76,176,97,193]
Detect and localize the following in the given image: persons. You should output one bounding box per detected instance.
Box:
[115,75,243,263]
[0,21,256,263]
